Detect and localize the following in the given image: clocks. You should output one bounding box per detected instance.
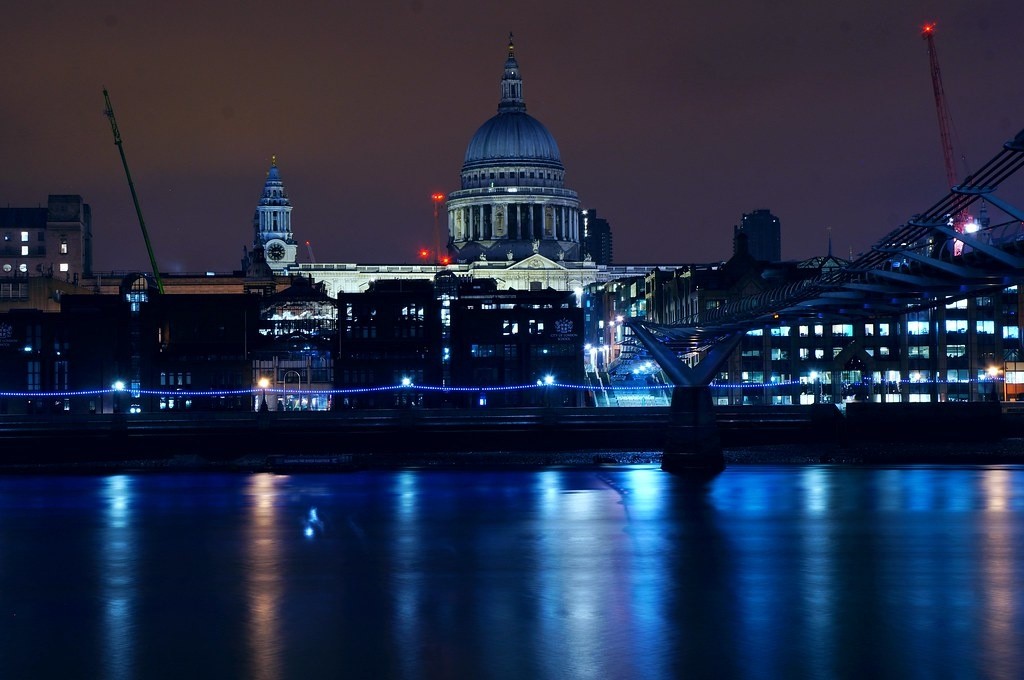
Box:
[267,243,285,260]
